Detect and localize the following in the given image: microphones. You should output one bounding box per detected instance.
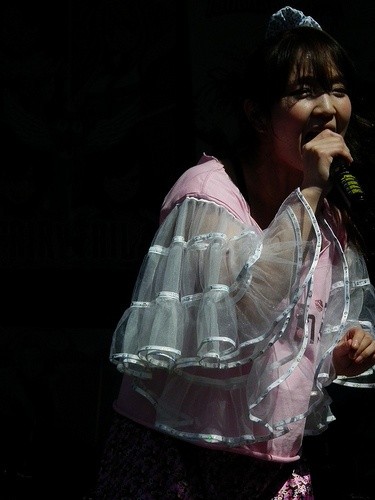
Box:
[330,156,366,209]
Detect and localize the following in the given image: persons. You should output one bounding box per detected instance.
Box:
[93,6,375,500]
